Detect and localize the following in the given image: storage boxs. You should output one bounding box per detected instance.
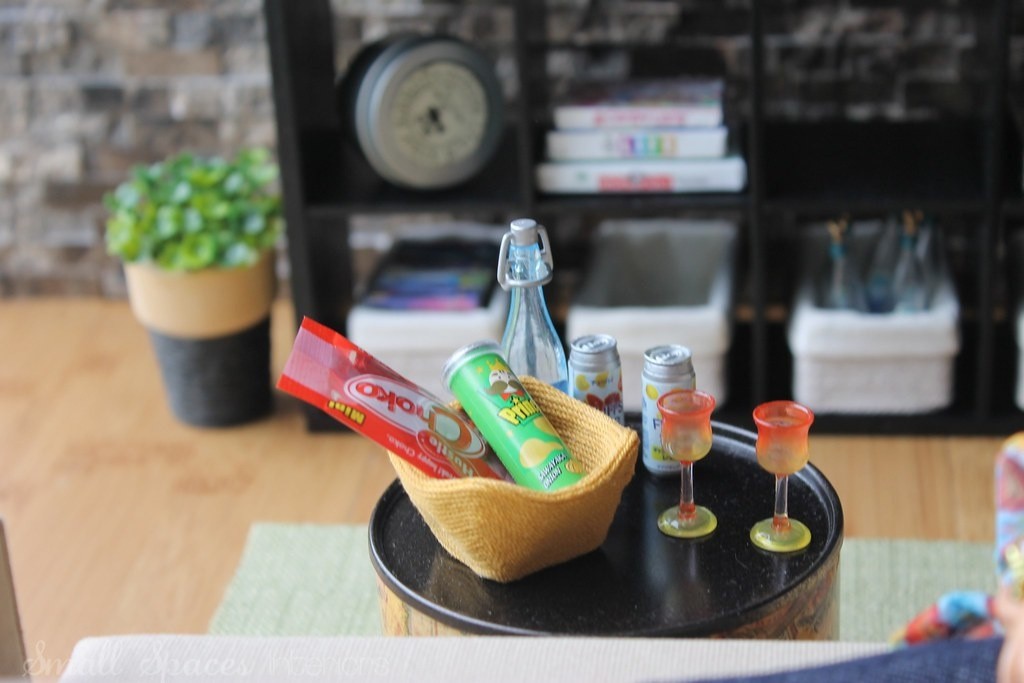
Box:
[778,218,962,419]
[344,214,507,407]
[564,216,743,412]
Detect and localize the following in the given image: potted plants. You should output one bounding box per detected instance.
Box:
[96,142,284,429]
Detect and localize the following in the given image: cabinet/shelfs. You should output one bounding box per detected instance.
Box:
[258,1,1023,441]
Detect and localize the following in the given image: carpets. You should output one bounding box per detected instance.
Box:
[206,520,996,639]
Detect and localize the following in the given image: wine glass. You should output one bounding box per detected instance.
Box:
[656,389,718,540]
[749,398,816,552]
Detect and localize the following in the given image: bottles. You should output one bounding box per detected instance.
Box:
[495,220,567,394]
